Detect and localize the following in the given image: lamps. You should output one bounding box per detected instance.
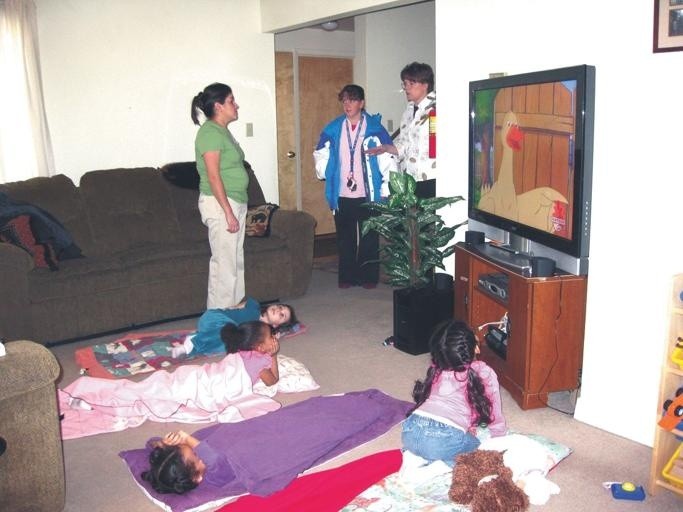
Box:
[322,21,339,31]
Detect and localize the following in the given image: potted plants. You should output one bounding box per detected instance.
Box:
[360,172,468,355]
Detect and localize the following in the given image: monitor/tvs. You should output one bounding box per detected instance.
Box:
[468,67,595,269]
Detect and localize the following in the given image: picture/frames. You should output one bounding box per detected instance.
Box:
[653,0,683,52]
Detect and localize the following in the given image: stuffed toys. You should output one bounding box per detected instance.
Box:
[448,449,530,512]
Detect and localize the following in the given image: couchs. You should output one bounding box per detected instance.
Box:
[0,161,317,346]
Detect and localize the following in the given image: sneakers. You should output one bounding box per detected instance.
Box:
[362,280,376,288]
[339,281,351,288]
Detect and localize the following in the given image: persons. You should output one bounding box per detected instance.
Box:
[191,82,250,310]
[396,320,507,494]
[144,430,233,494]
[221,321,280,387]
[313,84,396,289]
[172,299,297,358]
[364,62,435,202]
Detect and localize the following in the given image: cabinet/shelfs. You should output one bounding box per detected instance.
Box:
[450,243,587,410]
[648,272,683,497]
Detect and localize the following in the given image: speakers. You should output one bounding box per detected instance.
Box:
[532,257,556,277]
[465,231,484,243]
